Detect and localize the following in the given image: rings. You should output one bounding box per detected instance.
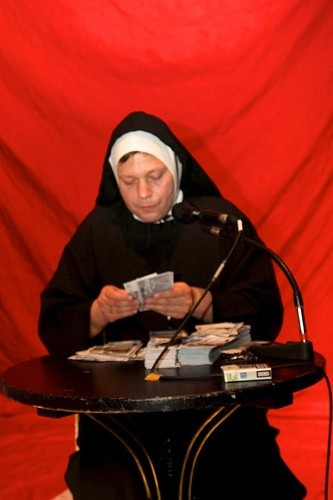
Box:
[108,306,114,314]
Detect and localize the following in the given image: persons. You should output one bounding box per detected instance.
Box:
[37,110,310,500]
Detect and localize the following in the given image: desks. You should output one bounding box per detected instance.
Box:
[1,348,326,500]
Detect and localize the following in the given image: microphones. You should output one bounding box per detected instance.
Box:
[171,202,245,231]
[202,224,313,364]
